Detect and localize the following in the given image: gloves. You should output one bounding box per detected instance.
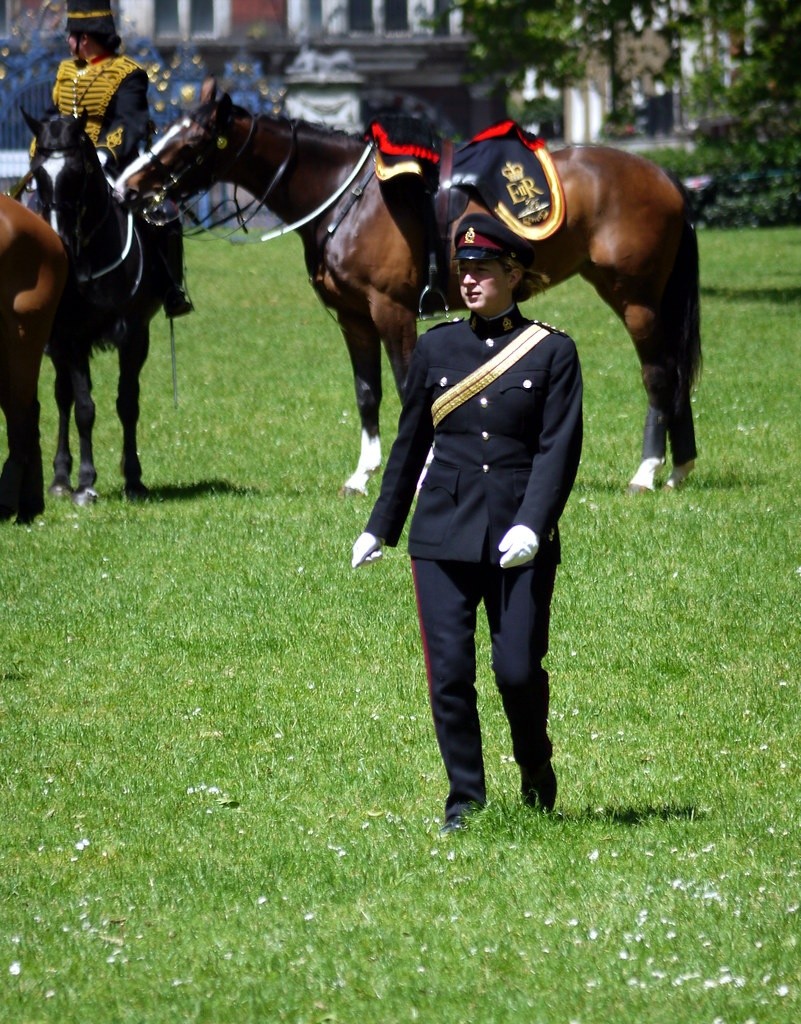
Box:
[351,531,384,569]
[497,524,540,569]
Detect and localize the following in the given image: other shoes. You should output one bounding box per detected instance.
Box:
[162,284,191,320]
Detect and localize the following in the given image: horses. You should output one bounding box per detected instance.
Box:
[20,102,185,505]
[112,75,704,502]
[0,191,109,527]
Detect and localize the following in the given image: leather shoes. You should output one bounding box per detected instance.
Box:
[439,817,468,837]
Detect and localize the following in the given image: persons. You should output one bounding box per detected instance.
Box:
[351,212,583,834]
[27,0,193,320]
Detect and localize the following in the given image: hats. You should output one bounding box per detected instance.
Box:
[63,0,115,35]
[451,212,533,279]
